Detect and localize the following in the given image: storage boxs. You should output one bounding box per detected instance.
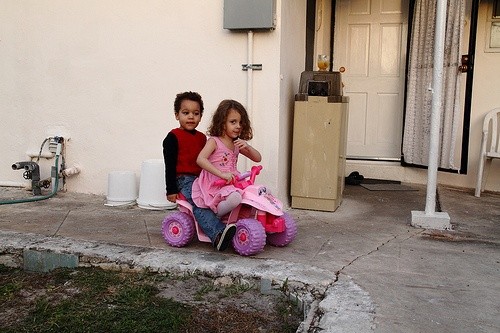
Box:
[223,0,277,31]
[104,169,139,208]
[136,159,178,210]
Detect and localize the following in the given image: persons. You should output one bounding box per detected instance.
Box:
[163,91,237,252]
[191,100,262,219]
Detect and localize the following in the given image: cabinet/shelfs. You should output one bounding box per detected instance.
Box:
[290,95,349,212]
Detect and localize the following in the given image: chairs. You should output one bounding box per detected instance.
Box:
[475,107,500,198]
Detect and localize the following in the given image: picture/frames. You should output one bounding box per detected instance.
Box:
[484,21,500,53]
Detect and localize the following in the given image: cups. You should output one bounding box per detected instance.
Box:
[318,54,328,71]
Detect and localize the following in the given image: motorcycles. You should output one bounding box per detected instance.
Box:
[160,165,297,256]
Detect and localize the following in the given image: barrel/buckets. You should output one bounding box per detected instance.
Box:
[105,171,137,207]
[136,159,177,210]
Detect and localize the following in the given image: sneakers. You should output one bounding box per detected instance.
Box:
[214,223,238,251]
